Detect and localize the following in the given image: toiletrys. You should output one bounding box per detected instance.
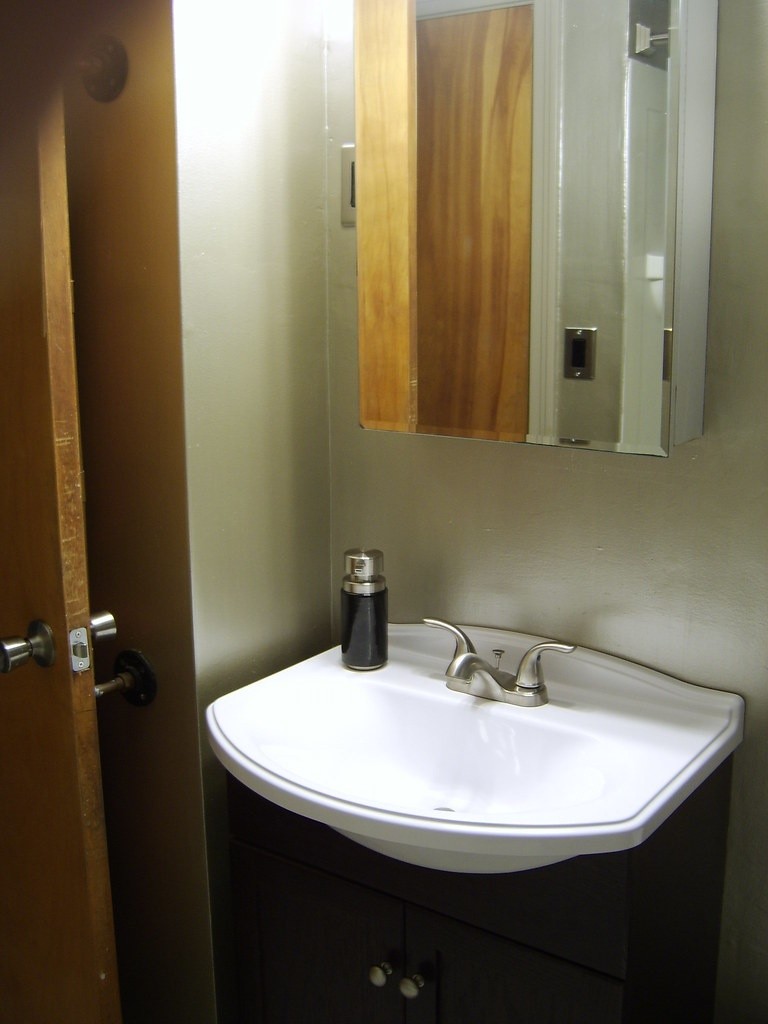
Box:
[338,546,388,672]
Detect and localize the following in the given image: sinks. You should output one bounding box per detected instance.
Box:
[205,676,697,876]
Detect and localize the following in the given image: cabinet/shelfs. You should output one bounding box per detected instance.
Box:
[221,755,734,1024]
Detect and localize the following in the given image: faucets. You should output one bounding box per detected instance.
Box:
[443,649,509,702]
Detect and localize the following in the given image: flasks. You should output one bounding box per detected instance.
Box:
[339,546,390,672]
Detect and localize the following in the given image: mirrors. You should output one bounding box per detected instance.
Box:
[353,1,687,458]
